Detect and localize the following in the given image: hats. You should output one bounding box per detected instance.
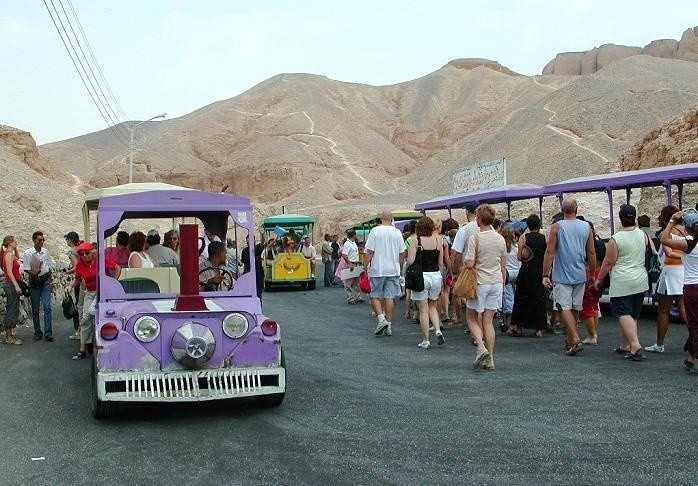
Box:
[359,271,370,292]
[618,203,635,217]
[521,244,533,262]
[461,201,477,213]
[64,231,79,241]
[681,207,697,228]
[637,214,650,226]
[147,228,158,236]
[76,242,94,252]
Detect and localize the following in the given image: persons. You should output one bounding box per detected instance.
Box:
[318,197,696,377]
[0,235,24,346]
[21,230,56,342]
[60,228,317,363]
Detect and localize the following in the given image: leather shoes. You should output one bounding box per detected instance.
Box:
[46,335,53,340]
[34,335,40,340]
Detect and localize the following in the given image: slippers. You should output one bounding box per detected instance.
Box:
[623,351,646,361]
[613,345,629,353]
[530,332,543,337]
[506,329,520,336]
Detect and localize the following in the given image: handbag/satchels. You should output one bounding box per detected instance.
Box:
[17,280,31,296]
[62,290,76,319]
[643,230,660,282]
[404,235,424,291]
[594,233,605,259]
[450,233,478,299]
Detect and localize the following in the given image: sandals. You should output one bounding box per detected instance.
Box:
[72,351,85,360]
[564,337,571,350]
[564,340,583,355]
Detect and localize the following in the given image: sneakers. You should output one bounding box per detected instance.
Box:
[68,332,80,339]
[6,336,23,344]
[581,337,596,343]
[478,356,494,369]
[373,318,389,335]
[311,273,314,278]
[644,343,663,352]
[382,327,392,336]
[12,335,22,341]
[417,341,430,348]
[435,330,444,345]
[472,345,489,368]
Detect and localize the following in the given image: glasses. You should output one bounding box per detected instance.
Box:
[36,237,44,242]
[79,251,90,255]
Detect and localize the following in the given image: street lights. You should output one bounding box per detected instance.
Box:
[128,110,167,184]
